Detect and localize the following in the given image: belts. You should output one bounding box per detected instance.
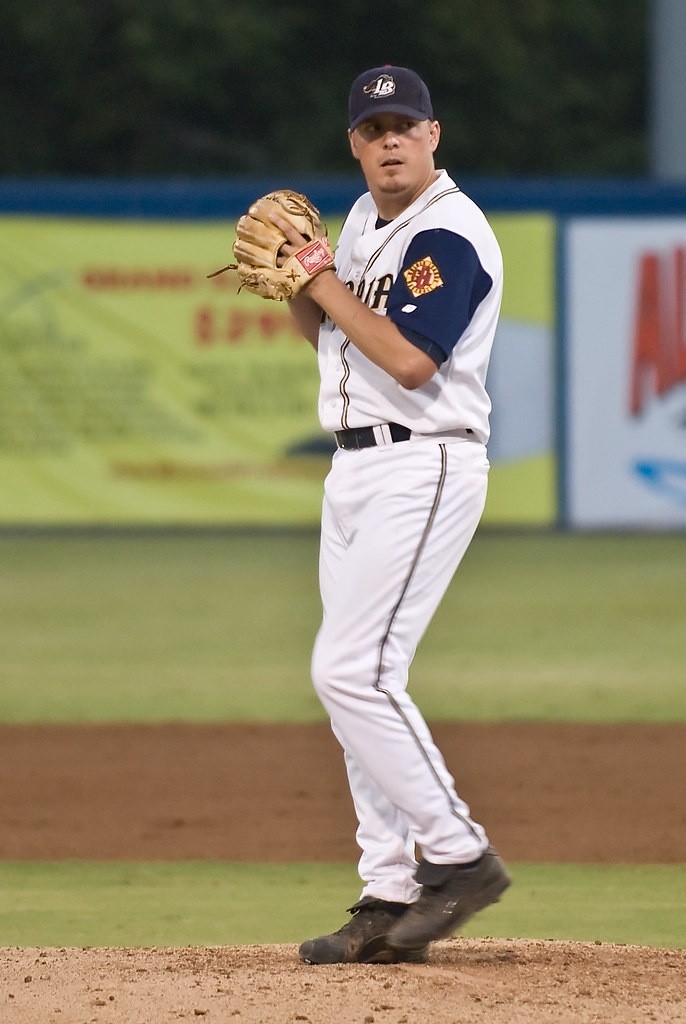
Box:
[334,421,473,450]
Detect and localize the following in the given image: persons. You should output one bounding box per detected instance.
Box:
[207,66,513,963]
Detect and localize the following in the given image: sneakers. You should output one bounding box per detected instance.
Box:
[387,845,512,952]
[299,897,431,965]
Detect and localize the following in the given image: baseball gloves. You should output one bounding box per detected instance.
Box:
[232,189,337,302]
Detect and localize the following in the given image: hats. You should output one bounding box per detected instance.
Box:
[348,65,433,134]
